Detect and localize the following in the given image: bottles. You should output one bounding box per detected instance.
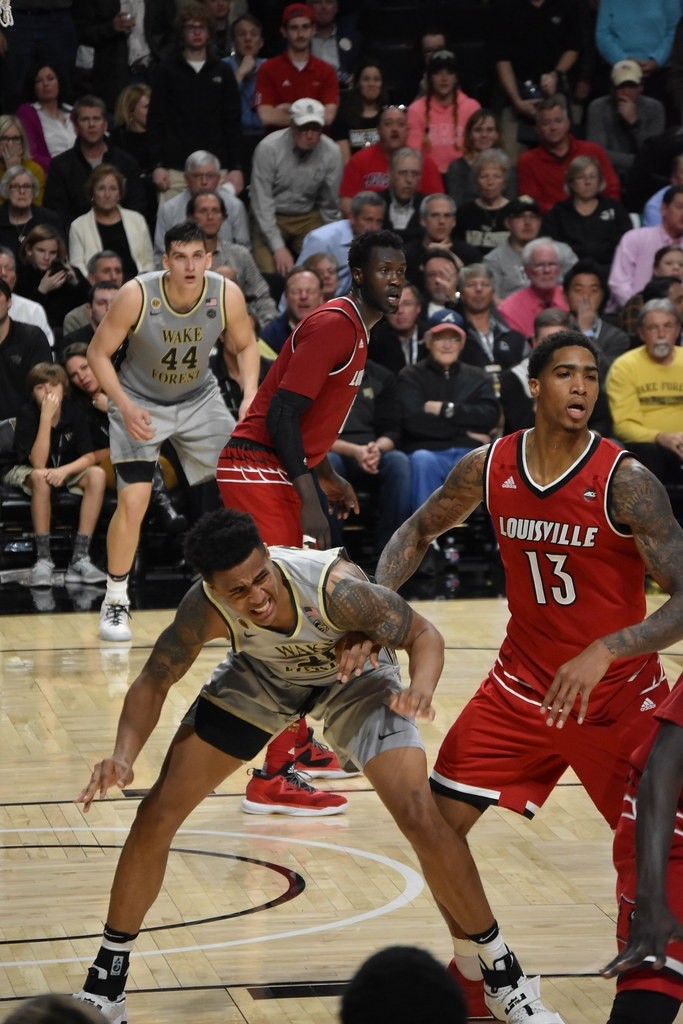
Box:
[443,537,459,600]
[121,0,133,21]
[525,80,541,99]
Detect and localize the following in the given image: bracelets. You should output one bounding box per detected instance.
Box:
[377,447,385,456]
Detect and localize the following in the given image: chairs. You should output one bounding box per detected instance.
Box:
[0,417,369,549]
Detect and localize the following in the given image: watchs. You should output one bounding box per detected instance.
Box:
[444,403,455,419]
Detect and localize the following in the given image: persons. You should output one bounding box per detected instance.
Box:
[598,671,683,1023]
[331,330,683,1024]
[86,222,261,642]
[339,944,470,1024]
[215,229,407,818]
[0,0,683,587]
[67,508,564,1024]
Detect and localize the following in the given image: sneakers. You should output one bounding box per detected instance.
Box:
[29,558,55,584]
[67,584,106,610]
[74,963,128,1024]
[243,759,348,817]
[262,725,364,779]
[66,558,107,583]
[98,602,132,639]
[100,641,130,690]
[482,952,570,1024]
[31,588,56,611]
[447,959,502,1023]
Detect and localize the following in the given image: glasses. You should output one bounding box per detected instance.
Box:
[1,136,21,143]
[383,105,407,111]
[10,183,32,192]
[186,24,209,33]
[532,262,558,272]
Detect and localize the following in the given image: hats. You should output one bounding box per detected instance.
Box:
[431,51,460,75]
[506,194,543,218]
[611,59,643,88]
[424,310,468,349]
[290,98,326,128]
[284,5,314,23]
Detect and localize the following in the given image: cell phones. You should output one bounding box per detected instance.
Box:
[48,258,63,277]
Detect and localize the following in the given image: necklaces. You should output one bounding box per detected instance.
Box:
[10,210,29,243]
[354,104,380,148]
[480,196,503,228]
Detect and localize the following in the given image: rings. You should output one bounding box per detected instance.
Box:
[93,401,96,405]
[676,442,683,449]
